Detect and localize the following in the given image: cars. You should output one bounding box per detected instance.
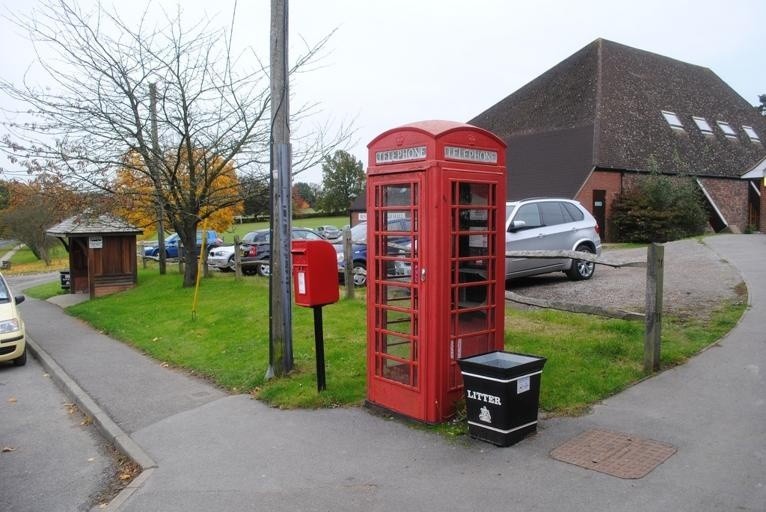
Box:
[0,272,27,365]
[330,217,417,287]
[206,245,237,273]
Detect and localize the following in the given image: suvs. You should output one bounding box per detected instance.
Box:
[315,225,341,239]
[238,227,332,279]
[143,230,223,262]
[394,196,602,301]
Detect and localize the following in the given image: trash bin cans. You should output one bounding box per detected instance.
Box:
[60,271,71,289]
[456,352,548,447]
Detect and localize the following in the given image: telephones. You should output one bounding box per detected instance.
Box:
[462,210,468,230]
